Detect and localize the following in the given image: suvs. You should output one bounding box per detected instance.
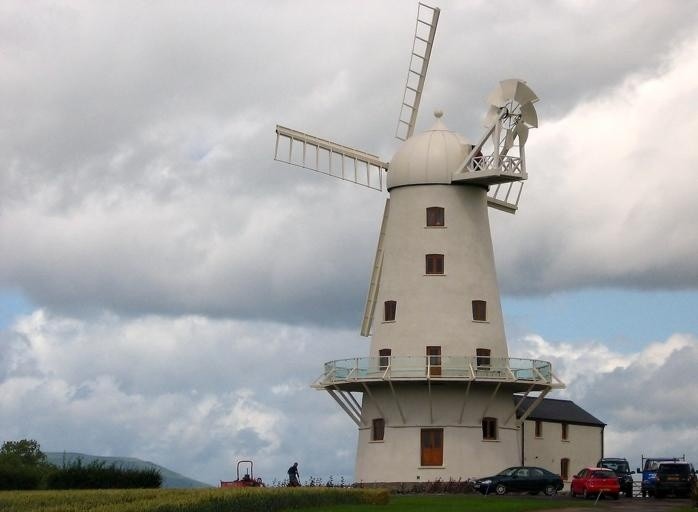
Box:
[597,457,635,497]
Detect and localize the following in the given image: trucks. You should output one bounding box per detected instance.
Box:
[637,454,685,497]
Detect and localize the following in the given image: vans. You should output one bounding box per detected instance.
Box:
[655,462,698,498]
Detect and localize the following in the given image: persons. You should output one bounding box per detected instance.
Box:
[286,462,301,486]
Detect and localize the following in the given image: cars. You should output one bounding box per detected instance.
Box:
[570,467,621,499]
[473,466,564,496]
